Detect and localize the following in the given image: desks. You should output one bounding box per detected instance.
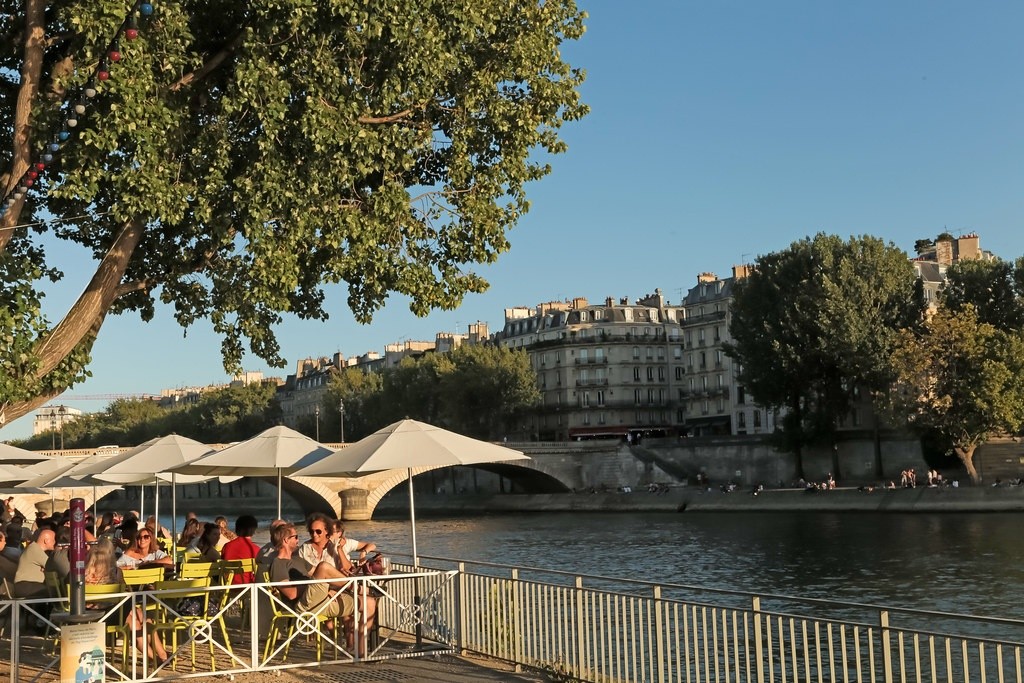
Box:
[352,571,412,662]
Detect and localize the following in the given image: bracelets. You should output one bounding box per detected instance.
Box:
[363,549,368,555]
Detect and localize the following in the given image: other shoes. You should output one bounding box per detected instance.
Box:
[35,626,57,636]
[163,660,172,668]
[359,656,384,664]
[368,586,387,597]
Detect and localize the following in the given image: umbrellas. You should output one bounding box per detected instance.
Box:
[0,414,532,591]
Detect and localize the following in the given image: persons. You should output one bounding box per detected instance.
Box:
[0,497,378,658]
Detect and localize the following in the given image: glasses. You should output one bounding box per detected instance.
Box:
[308,529,328,534]
[137,534,150,541]
[289,534,298,540]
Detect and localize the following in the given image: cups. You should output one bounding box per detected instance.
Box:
[380,557,391,575]
[128,559,135,569]
[116,561,127,570]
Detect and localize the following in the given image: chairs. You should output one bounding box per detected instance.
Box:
[3,537,341,681]
